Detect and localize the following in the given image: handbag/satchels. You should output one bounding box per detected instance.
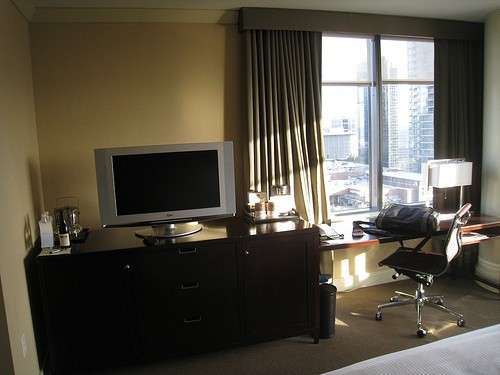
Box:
[352,204,438,251]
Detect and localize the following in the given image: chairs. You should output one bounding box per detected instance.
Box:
[376,203,471,337]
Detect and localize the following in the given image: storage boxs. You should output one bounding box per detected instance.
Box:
[39,217,53,248]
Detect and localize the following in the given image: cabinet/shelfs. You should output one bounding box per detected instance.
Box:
[36,218,318,375]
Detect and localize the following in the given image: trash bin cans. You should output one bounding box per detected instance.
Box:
[319,284,337,339]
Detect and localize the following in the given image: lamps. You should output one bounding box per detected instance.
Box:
[428,161,474,207]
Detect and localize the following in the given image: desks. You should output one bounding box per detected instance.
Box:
[318,209,500,252]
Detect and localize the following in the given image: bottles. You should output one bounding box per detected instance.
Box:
[58,209,71,249]
[255,203,267,220]
[38,211,54,252]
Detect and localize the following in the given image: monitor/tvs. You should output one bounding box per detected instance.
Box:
[94,141,236,238]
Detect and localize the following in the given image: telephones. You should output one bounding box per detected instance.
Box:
[312,223,339,240]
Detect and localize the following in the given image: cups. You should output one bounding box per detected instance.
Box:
[56,204,79,234]
